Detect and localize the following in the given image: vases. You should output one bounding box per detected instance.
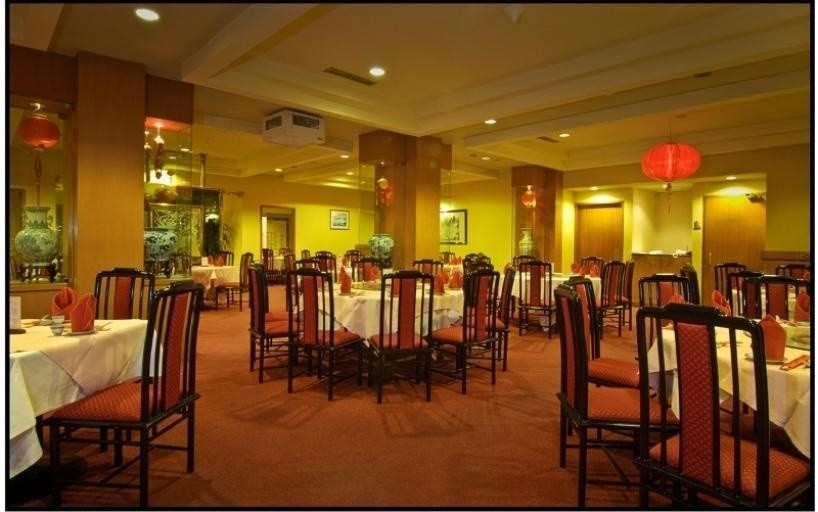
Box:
[518,227,533,256]
[144,227,177,263]
[14,206,62,268]
[369,234,394,259]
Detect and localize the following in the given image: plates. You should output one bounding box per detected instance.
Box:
[41,319,70,323]
[746,353,788,364]
[336,292,355,295]
[61,330,97,335]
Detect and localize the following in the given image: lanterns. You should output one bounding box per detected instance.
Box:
[17,113,60,206]
[522,191,536,224]
[642,143,702,216]
[374,177,392,207]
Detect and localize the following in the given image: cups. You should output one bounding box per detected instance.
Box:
[50,327,63,337]
[52,315,65,324]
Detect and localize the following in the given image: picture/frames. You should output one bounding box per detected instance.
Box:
[330,209,350,230]
[439,209,467,245]
